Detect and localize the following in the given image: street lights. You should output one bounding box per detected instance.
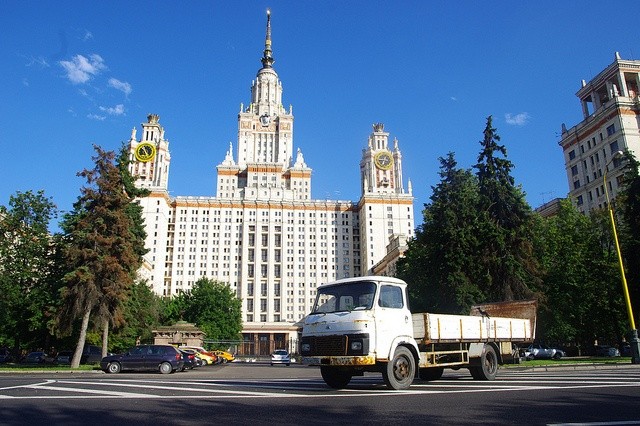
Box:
[603,150,639,365]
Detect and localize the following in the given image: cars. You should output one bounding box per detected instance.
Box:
[0,348,10,362]
[519,344,565,360]
[26,351,45,362]
[80,344,116,364]
[271,349,291,367]
[174,344,236,369]
[44,354,57,364]
[55,350,74,364]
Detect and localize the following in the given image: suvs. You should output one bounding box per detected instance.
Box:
[101,345,184,374]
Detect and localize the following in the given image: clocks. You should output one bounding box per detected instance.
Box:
[135,143,156,162]
[375,151,394,170]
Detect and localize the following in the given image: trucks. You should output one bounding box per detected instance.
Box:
[301,275,539,390]
